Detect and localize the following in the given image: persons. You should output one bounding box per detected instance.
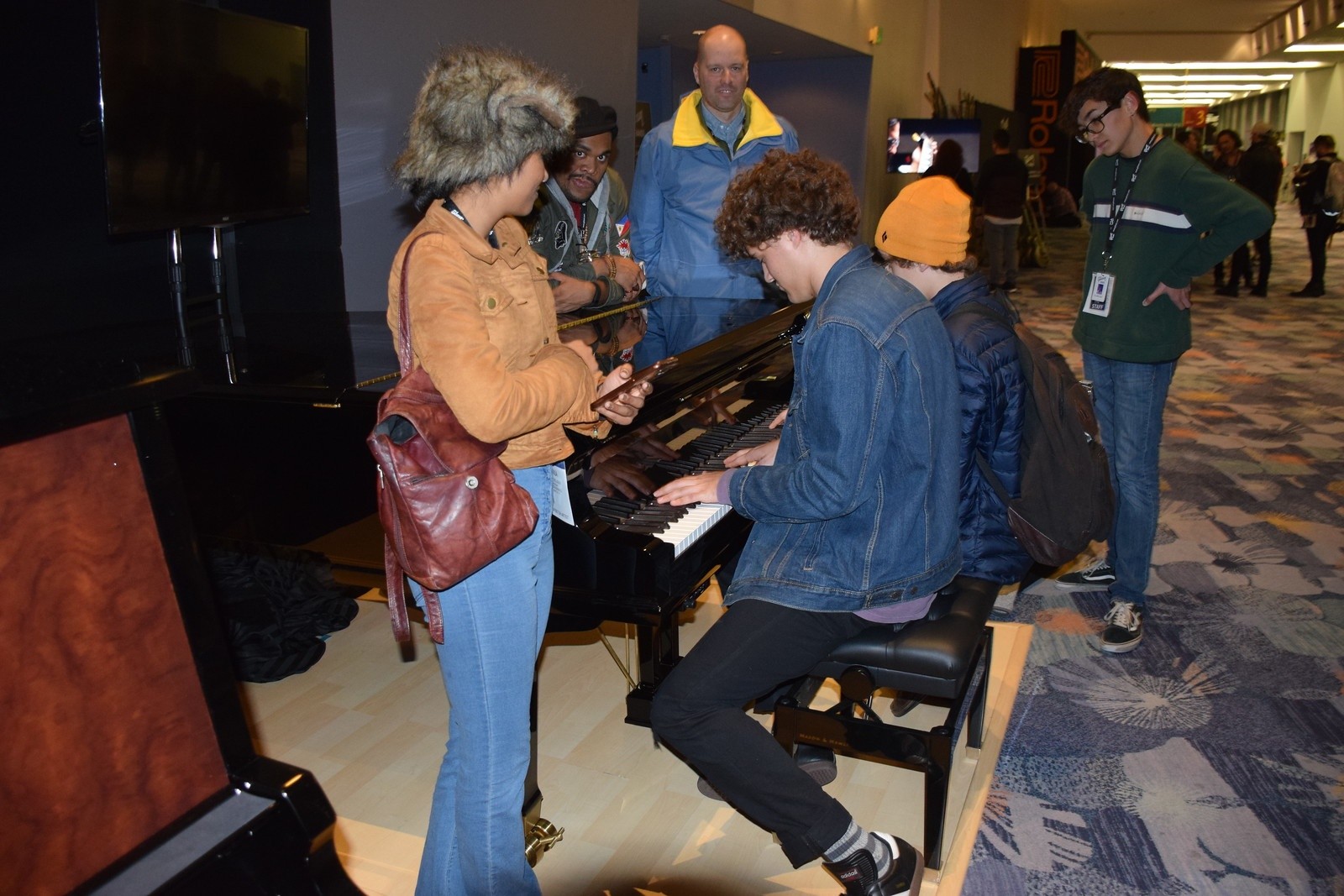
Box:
[873,174,1042,716]
[628,25,801,300]
[515,95,647,315]
[1175,122,1338,298]
[1054,67,1275,651]
[558,304,647,377]
[924,129,1080,292]
[694,391,740,426]
[386,45,653,896]
[635,297,792,374]
[649,148,966,896]
[889,120,937,173]
[585,436,682,503]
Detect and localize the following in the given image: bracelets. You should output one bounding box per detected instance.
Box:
[587,281,601,308]
[605,334,619,358]
[601,253,617,280]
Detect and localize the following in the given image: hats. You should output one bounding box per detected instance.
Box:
[874,176,971,267]
[1250,120,1276,138]
[571,96,617,138]
[393,40,579,188]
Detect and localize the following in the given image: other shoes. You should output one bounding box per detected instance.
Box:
[1250,285,1267,297]
[1215,286,1237,297]
[1246,281,1256,290]
[889,690,927,717]
[1291,285,1325,298]
[989,283,997,293]
[1001,281,1017,293]
[1214,278,1222,288]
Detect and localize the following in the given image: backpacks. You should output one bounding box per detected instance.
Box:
[941,288,1115,566]
[367,231,539,663]
[1317,155,1344,212]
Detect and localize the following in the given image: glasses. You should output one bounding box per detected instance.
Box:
[1074,88,1131,144]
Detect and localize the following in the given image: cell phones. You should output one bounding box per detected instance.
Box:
[590,355,681,412]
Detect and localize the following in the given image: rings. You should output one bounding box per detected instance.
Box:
[626,315,640,322]
[625,290,633,293]
[632,283,640,292]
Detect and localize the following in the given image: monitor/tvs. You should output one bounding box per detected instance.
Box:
[885,117,982,175]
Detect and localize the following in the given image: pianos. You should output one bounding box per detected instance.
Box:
[177,292,807,732]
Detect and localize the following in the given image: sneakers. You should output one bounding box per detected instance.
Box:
[1100,601,1143,653]
[697,738,837,802]
[821,831,925,896]
[1055,555,1116,587]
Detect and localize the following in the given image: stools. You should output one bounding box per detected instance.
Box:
[772,576,1002,870]
